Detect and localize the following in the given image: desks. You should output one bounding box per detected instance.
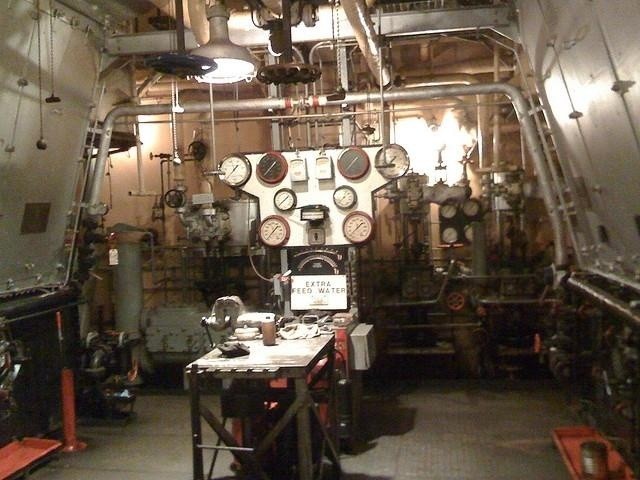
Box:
[185,328,341,480]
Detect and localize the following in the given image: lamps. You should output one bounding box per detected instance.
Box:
[186,5,260,84]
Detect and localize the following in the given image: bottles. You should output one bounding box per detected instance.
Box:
[262,316,276,346]
